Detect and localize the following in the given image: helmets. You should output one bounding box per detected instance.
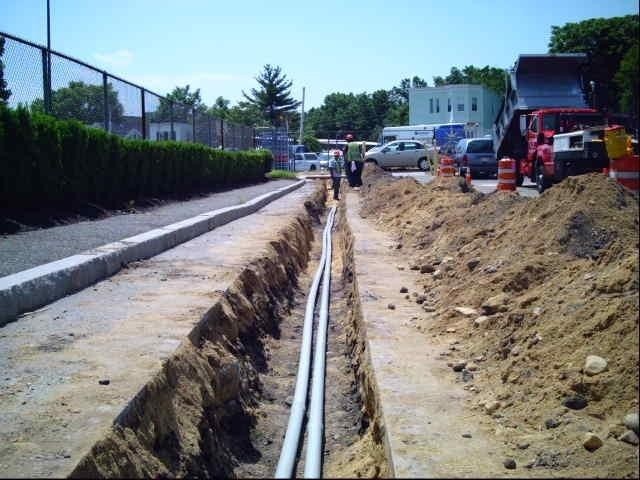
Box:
[334,151,341,156]
[345,133,353,141]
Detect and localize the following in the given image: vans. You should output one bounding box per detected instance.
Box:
[317,152,333,168]
[290,152,321,172]
[454,138,498,181]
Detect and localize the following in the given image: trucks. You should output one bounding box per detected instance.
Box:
[553,125,638,188]
[489,49,607,194]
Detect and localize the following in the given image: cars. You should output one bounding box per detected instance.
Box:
[291,145,305,153]
[359,139,441,173]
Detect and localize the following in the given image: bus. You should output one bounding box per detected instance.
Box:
[317,139,380,158]
[381,123,471,157]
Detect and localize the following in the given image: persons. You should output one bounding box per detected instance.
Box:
[328,134,364,201]
